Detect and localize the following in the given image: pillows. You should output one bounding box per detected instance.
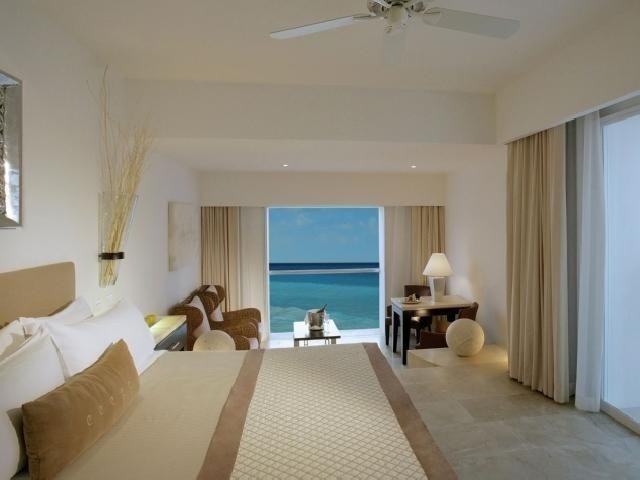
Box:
[0,294,158,480]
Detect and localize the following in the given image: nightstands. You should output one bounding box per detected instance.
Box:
[149,314,188,351]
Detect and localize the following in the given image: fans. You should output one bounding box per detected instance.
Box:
[268,0,520,42]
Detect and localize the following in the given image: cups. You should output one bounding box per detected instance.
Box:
[403,297,419,302]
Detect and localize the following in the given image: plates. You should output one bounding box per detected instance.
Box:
[400,301,420,304]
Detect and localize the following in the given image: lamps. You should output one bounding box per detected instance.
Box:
[423,252,453,301]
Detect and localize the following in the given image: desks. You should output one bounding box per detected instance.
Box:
[390,296,472,365]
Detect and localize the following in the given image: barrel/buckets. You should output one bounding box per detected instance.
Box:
[307,309,325,332]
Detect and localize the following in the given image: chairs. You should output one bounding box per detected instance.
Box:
[385,283,479,350]
[168,285,262,350]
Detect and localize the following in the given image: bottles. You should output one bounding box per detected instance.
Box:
[316,304,328,313]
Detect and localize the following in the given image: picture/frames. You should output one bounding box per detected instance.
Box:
[0,70,23,228]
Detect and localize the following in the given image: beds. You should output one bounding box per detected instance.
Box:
[0,261,459,480]
